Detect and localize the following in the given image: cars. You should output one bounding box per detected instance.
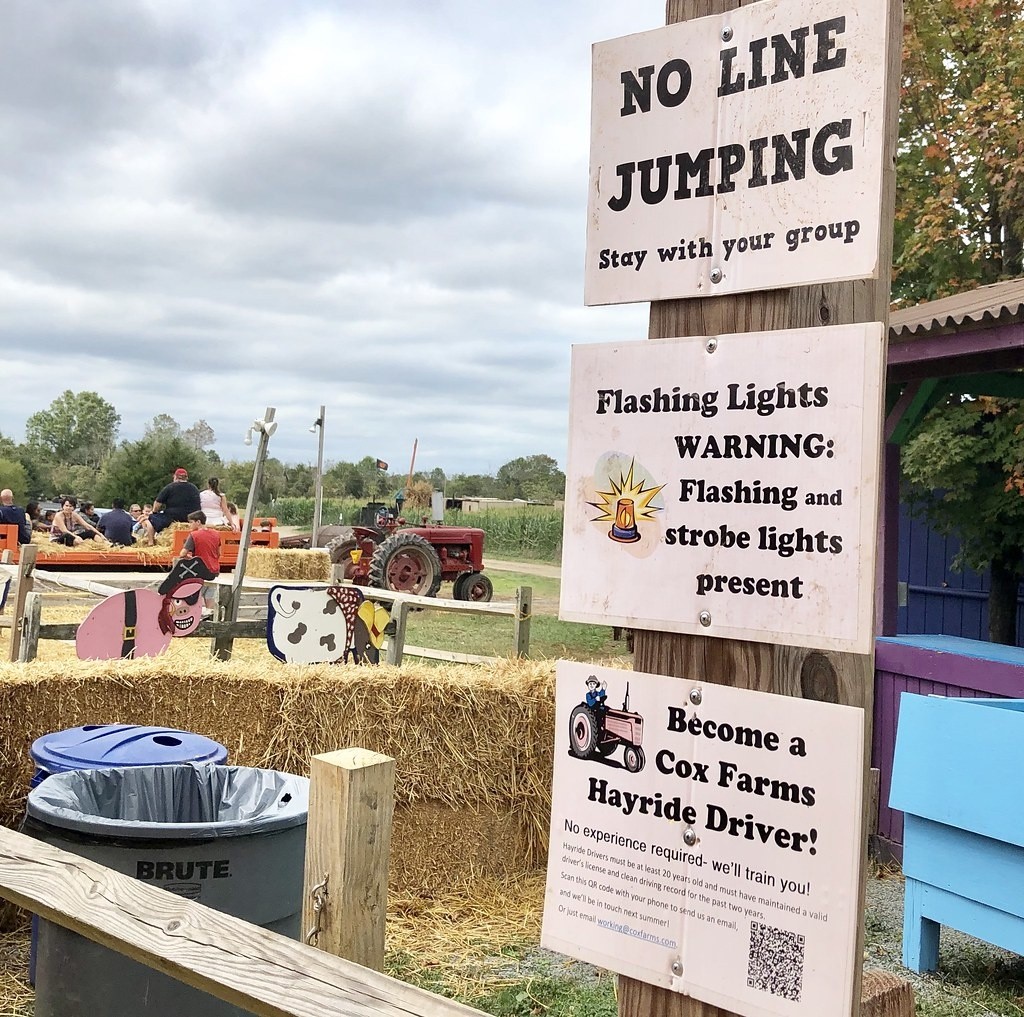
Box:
[73,508,135,525]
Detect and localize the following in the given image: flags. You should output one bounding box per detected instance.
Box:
[377,460,388,470]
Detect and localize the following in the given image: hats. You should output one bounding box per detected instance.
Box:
[175,469,187,475]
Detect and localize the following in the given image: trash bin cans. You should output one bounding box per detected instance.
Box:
[26,764,311,1017]
[32,726,229,786]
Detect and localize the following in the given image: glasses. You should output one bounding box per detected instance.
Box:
[132,510,141,512]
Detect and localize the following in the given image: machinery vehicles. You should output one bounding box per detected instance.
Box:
[323,505,494,612]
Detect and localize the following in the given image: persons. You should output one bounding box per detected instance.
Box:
[0,469,240,548]
[179,510,222,578]
